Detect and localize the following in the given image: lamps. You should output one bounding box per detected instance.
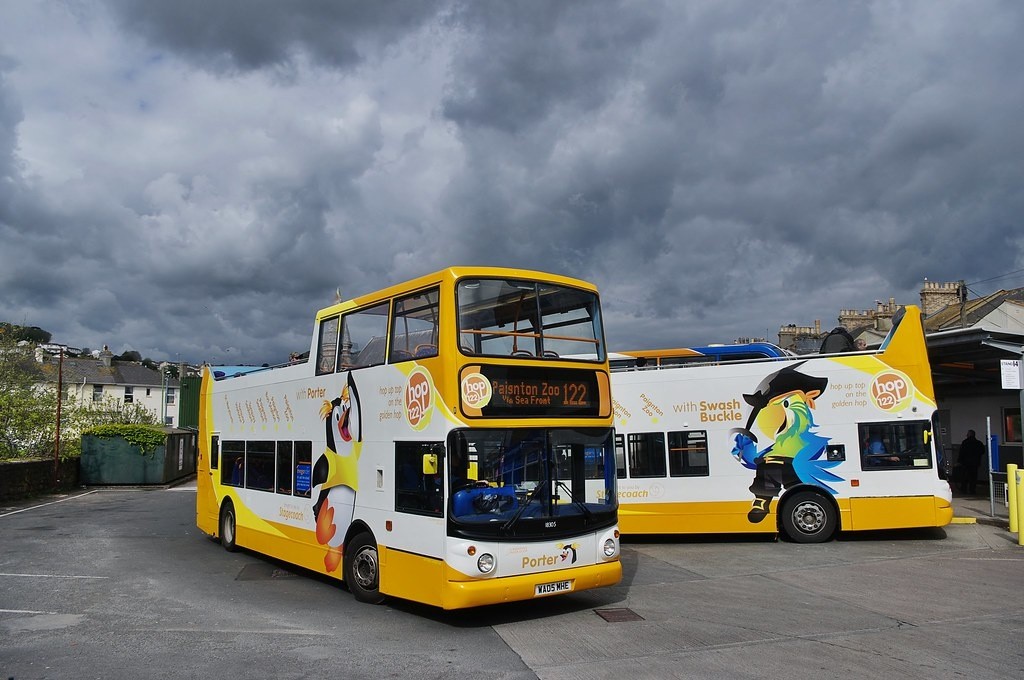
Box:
[183,383,187,386]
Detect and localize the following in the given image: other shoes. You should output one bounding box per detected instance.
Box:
[970,490,977,495]
[958,490,965,494]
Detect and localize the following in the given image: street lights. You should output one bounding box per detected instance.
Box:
[42,347,79,494]
[163,370,174,425]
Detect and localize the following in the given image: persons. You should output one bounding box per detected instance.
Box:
[958,429,987,496]
[862,426,901,467]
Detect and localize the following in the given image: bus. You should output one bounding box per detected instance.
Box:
[467,305,955,544]
[195,267,623,612]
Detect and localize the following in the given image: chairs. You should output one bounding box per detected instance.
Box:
[232,458,244,485]
[543,350,559,358]
[510,350,533,357]
[414,344,438,359]
[389,348,417,363]
[461,346,476,355]
